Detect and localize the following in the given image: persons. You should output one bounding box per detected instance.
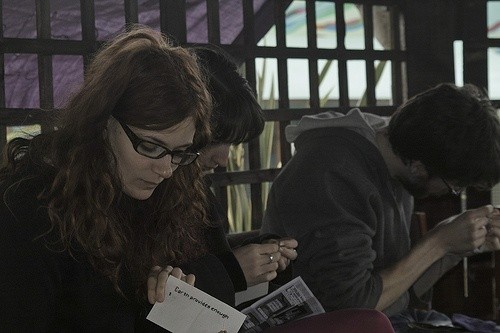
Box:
[186,44,395,333]
[267,82,500,333]
[0,28,212,333]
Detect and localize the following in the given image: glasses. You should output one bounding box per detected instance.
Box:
[112,113,201,165]
[442,177,467,197]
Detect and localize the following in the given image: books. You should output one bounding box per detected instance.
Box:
[241,276,326,330]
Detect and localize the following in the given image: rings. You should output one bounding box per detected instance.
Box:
[266,253,274,264]
[163,269,171,273]
